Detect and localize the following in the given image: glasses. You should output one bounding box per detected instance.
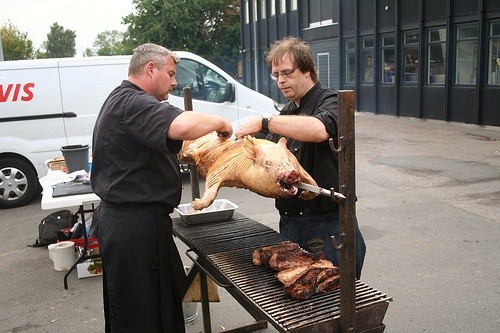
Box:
[270,67,299,81]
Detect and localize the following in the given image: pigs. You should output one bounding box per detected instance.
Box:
[175,130,319,210]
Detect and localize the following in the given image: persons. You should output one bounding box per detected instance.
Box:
[364,56,374,82]
[90,43,233,333]
[235,37,366,280]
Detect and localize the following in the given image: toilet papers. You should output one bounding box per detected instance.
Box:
[52,242,75,271]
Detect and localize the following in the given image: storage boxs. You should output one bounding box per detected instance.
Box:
[76,239,102,279]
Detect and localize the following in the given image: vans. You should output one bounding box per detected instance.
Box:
[0,51,284,208]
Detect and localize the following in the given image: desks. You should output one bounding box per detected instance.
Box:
[40,168,101,290]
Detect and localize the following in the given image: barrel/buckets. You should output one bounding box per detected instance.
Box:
[182,267,197,321]
[60,147,89,174]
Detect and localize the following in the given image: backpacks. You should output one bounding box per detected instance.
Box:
[27,211,82,247]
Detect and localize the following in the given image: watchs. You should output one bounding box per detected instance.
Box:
[261,113,273,130]
